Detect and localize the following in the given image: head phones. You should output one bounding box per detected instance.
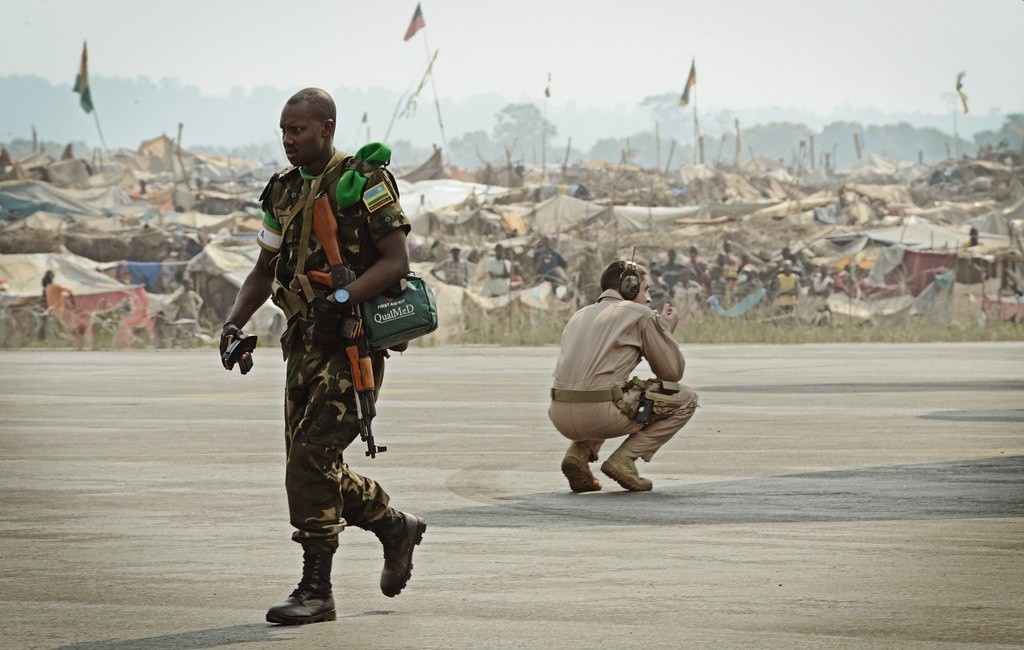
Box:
[619,261,641,300]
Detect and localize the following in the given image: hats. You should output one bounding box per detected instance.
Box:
[335,142,392,211]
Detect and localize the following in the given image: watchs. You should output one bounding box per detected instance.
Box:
[334,288,351,305]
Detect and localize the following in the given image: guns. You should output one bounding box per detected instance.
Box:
[303,193,389,459]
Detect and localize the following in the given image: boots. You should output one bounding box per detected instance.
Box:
[380,511,427,598]
[266,549,337,624]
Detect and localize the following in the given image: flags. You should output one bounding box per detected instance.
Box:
[404,4,425,41]
[679,62,696,105]
[956,73,969,114]
[71,42,94,114]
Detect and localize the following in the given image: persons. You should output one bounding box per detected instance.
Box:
[217,89,426,623]
[174,280,203,319]
[42,272,76,318]
[637,241,855,311]
[534,238,568,280]
[549,259,699,494]
[430,247,467,287]
[132,178,149,194]
[486,244,512,296]
[963,228,982,245]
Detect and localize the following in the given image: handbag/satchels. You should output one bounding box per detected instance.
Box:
[359,272,439,351]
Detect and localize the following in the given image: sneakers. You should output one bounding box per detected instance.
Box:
[601,447,652,491]
[561,442,602,492]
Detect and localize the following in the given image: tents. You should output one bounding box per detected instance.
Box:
[512,193,607,233]
[0,163,284,342]
[824,224,1004,298]
